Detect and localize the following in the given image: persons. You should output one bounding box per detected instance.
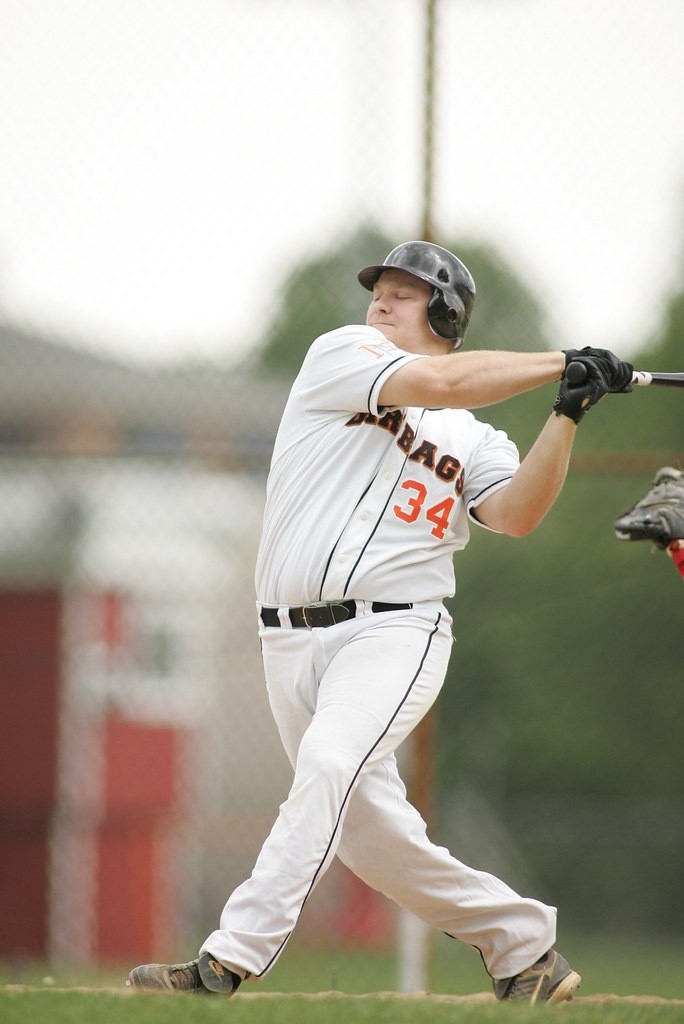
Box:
[129,242,632,1006]
[613,467,684,578]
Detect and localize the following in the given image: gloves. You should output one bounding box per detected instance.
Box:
[553,346,633,423]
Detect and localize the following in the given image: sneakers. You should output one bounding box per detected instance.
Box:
[129,952,241,999]
[493,948,582,1009]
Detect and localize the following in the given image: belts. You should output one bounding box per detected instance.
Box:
[260,599,413,630]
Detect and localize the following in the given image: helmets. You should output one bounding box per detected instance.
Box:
[357,241,476,350]
